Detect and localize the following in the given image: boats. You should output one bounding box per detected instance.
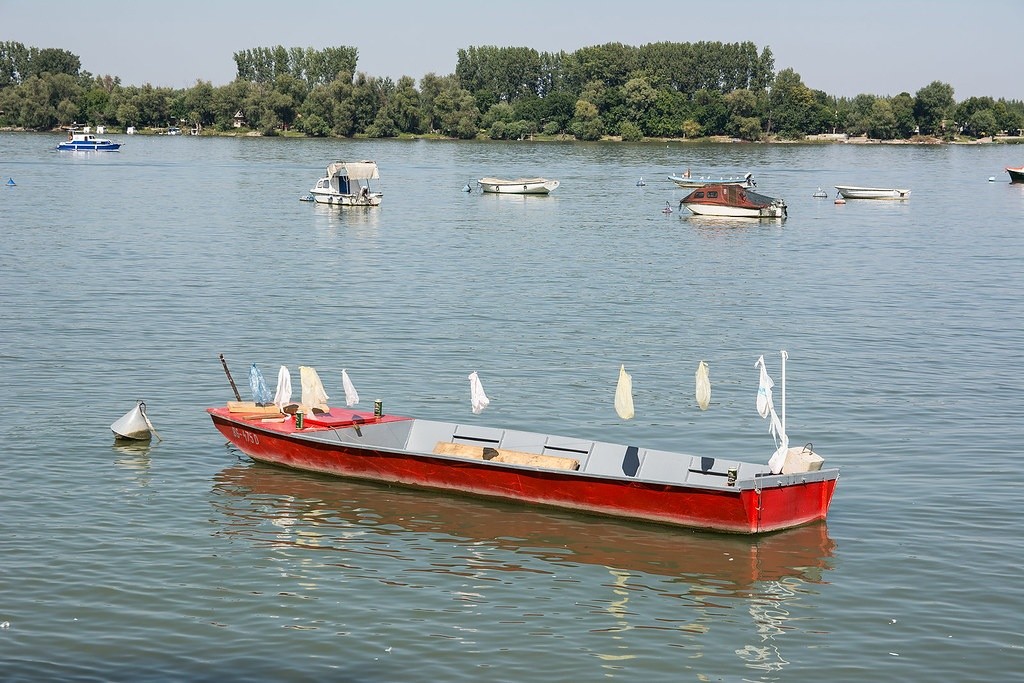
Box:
[679,185,788,219]
[205,356,842,535]
[310,160,384,206]
[56,130,125,153]
[666,168,758,189]
[476,177,560,195]
[834,185,911,200]
[1006,166,1024,184]
